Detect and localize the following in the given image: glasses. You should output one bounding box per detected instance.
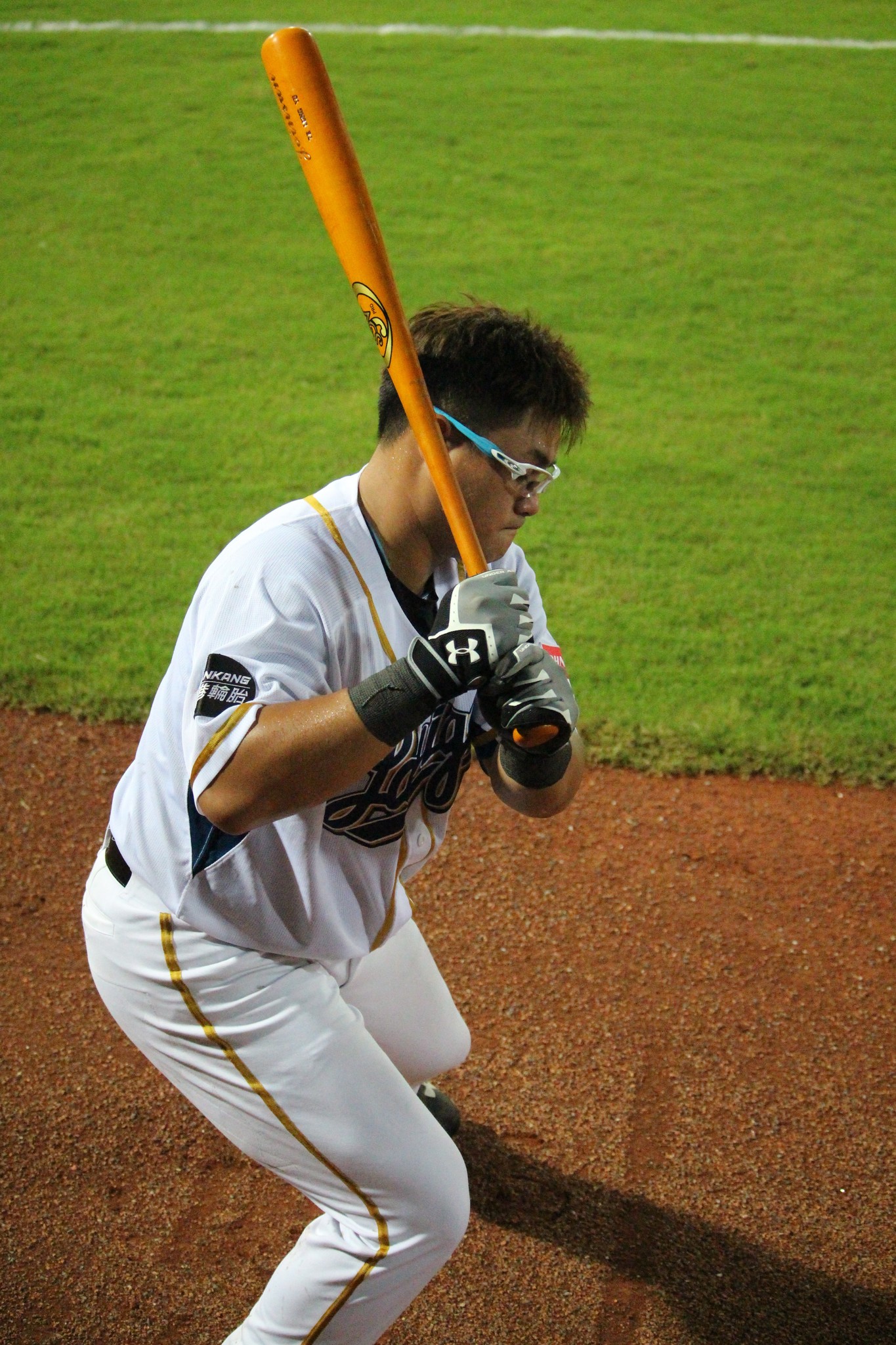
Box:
[434,406,561,498]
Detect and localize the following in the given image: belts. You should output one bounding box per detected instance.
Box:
[102,835,133,889]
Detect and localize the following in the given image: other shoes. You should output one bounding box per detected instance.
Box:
[417,1080,460,1133]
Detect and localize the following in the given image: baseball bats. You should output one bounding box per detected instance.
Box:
[259,24,560,751]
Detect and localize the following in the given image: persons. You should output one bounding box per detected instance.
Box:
[80,290,595,1345]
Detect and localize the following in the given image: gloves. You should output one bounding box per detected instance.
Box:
[406,565,537,702]
[483,645,579,760]
